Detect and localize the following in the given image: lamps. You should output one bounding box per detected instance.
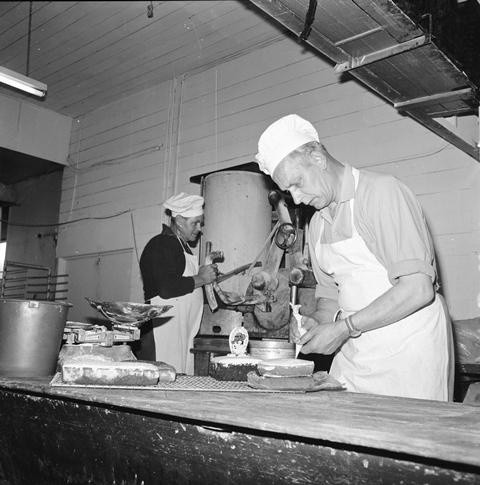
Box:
[0,65,48,97]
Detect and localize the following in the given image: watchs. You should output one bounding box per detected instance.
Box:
[345,318,360,338]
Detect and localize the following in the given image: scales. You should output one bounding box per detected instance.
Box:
[56,297,174,372]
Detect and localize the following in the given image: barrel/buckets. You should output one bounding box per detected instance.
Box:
[1,297,72,383]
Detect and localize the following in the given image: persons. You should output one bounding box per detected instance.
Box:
[255,113,458,404]
[139,193,218,375]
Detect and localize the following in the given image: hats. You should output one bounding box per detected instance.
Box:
[160,190,206,219]
[255,112,321,179]
